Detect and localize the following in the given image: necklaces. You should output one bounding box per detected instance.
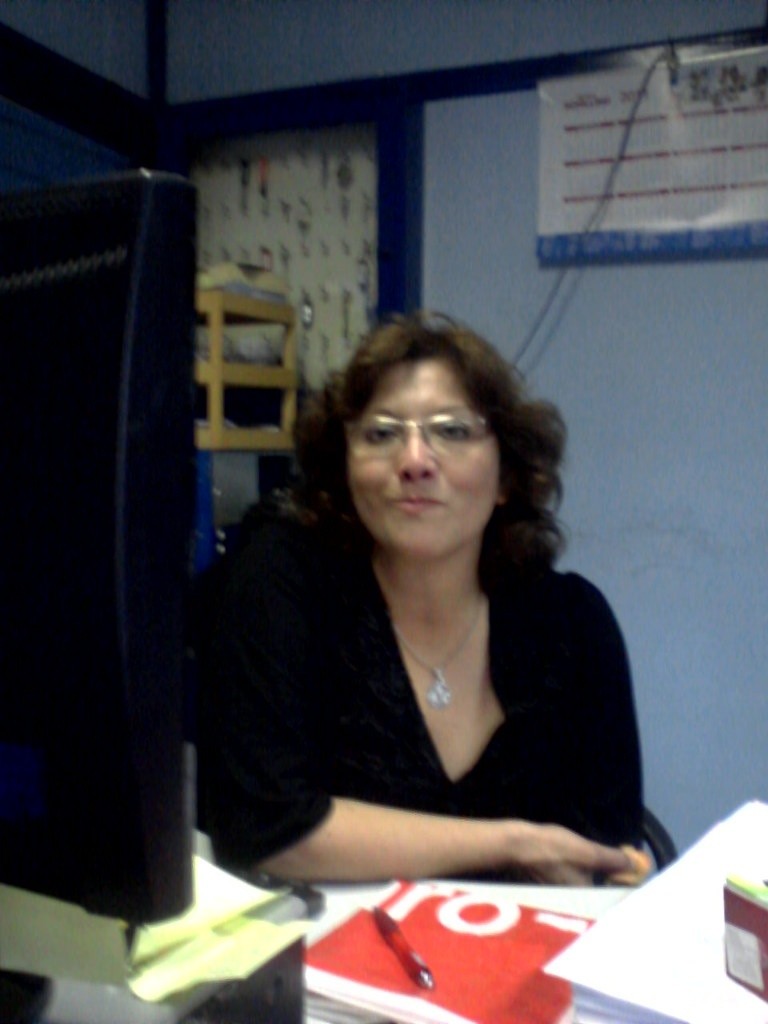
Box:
[388,592,487,710]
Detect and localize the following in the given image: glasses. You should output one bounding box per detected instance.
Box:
[343,413,487,459]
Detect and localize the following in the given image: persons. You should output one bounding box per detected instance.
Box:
[195,305,646,888]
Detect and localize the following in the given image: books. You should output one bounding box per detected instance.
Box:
[301,877,596,1024]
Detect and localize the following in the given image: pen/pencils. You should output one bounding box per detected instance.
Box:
[371,904,434,990]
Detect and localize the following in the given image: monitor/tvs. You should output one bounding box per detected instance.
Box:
[0,168,196,979]
